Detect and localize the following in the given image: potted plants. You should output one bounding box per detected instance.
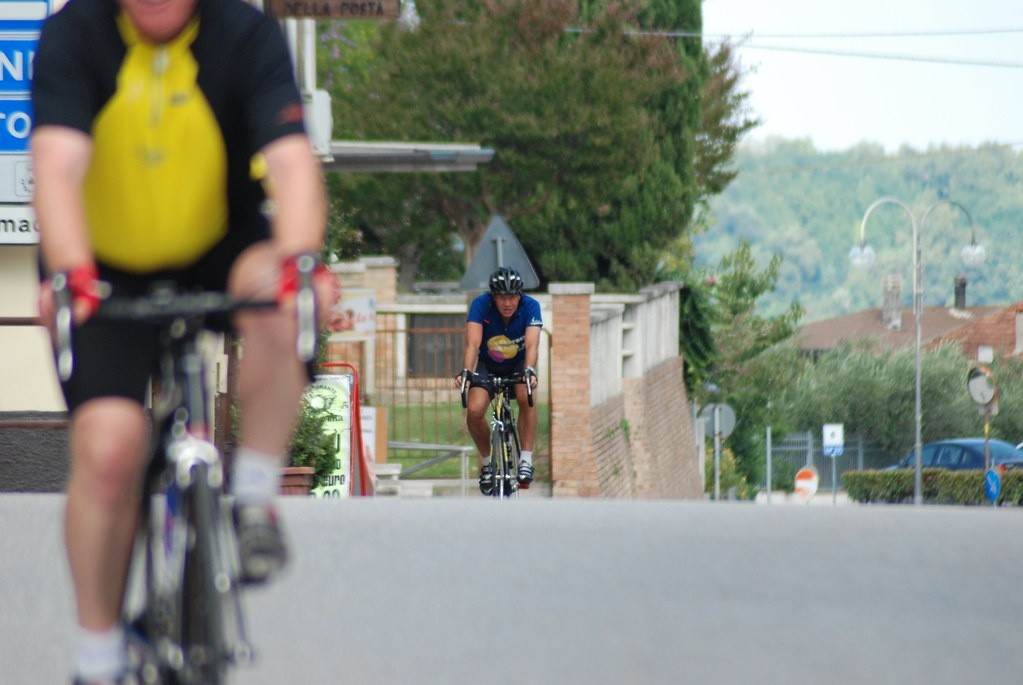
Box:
[229,387,337,495]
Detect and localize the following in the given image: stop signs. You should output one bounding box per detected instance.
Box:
[795,470,818,498]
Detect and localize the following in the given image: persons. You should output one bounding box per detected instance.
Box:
[455,266,544,496]
[28,0,342,685]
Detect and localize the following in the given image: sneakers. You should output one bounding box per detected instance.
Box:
[517,460,534,485]
[479,463,495,495]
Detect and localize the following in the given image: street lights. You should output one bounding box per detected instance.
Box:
[851,198,985,505]
[692,385,716,444]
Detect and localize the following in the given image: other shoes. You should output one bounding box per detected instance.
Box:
[233,497,283,582]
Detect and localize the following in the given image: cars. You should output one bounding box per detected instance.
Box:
[881,439,1023,471]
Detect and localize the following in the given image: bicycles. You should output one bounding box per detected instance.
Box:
[53,252,320,685]
[460,368,533,501]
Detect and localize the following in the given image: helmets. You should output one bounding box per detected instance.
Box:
[490,267,523,295]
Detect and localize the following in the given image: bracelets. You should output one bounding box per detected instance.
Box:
[527,366,537,375]
